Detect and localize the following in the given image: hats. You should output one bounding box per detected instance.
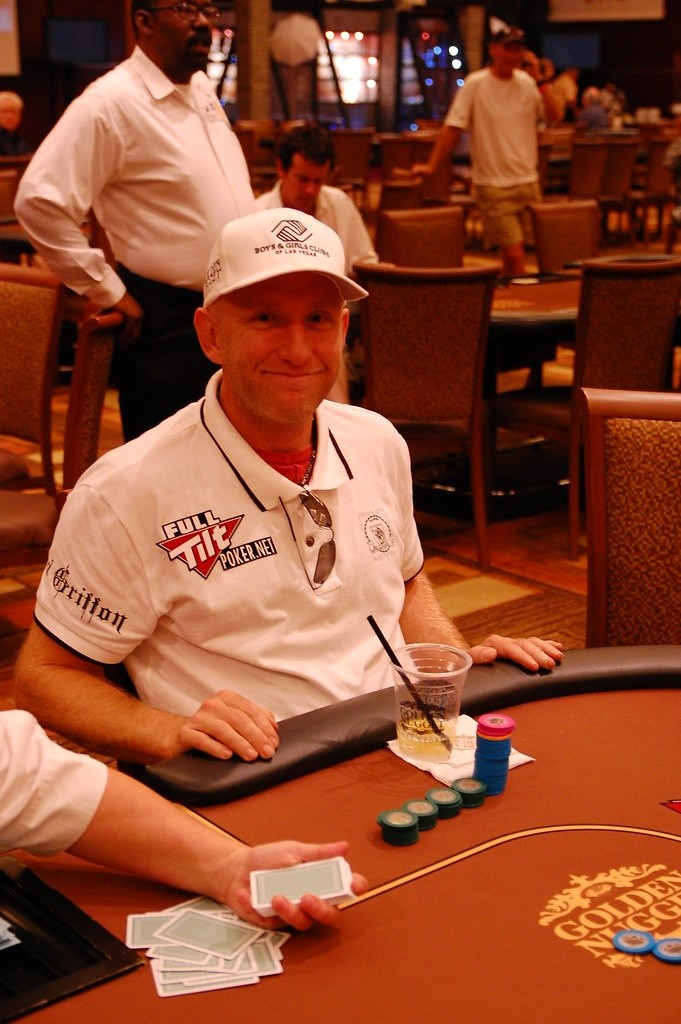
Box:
[203,208,369,309]
[496,26,525,46]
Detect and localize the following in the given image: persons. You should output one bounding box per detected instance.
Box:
[257,125,379,384]
[408,23,623,276]
[13,0,257,443]
[0,709,366,933]
[0,89,26,169]
[15,207,562,762]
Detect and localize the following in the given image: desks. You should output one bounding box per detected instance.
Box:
[0,647,680,1023]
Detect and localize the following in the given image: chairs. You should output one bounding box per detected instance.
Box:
[488,254,681,556]
[1,112,681,280]
[352,260,501,572]
[579,389,681,648]
[0,313,126,574]
[0,264,66,496]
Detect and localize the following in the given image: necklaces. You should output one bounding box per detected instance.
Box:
[300,423,319,484]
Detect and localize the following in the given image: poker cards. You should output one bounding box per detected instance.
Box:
[122,856,357,998]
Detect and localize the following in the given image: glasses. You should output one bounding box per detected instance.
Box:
[280,485,336,591]
[149,3,220,24]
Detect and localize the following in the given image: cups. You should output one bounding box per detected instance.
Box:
[389,643,473,758]
